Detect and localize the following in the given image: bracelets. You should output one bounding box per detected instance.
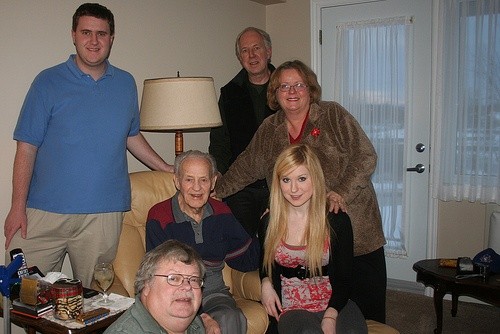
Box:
[322,316,337,322]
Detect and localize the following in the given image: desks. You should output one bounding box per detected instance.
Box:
[412,257,500,334]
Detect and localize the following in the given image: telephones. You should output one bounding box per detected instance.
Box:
[8,248,45,301]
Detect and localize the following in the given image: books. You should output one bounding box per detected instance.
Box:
[10,298,57,319]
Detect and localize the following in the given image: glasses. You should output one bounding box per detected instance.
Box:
[150,274,205,289]
[276,83,310,92]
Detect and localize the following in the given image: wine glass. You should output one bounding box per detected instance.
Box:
[94,262,115,306]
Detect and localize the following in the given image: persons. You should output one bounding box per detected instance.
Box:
[208,26,278,245]
[102,238,207,334]
[207,59,388,326]
[145,150,264,334]
[257,144,368,334]
[4,2,175,293]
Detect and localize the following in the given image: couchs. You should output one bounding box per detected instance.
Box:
[269,318,402,334]
[91,170,268,334]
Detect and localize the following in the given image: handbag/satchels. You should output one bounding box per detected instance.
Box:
[456,248,500,280]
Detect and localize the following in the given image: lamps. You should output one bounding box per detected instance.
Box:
[138,71,224,159]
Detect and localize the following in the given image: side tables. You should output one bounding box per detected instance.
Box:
[0,275,136,334]
